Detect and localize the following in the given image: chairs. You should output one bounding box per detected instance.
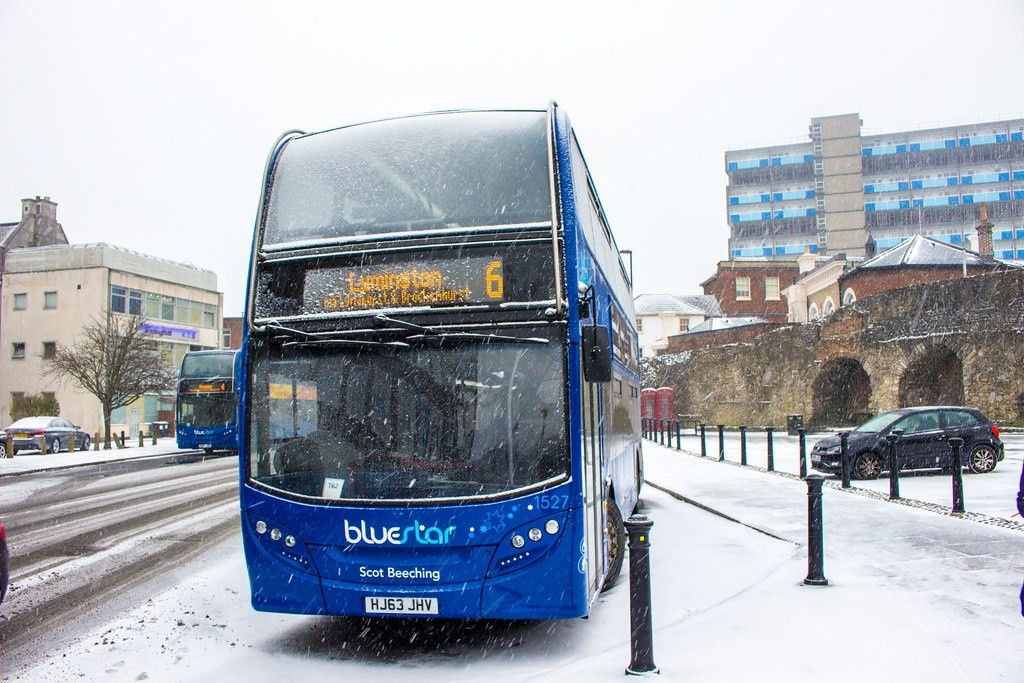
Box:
[924,416,938,429]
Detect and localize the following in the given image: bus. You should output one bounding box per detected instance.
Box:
[227,99,642,624]
[175,349,320,454]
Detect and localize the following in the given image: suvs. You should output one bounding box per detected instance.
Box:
[810,405,1005,479]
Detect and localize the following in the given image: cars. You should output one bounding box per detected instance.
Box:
[5,415,91,454]
[0,427,14,458]
